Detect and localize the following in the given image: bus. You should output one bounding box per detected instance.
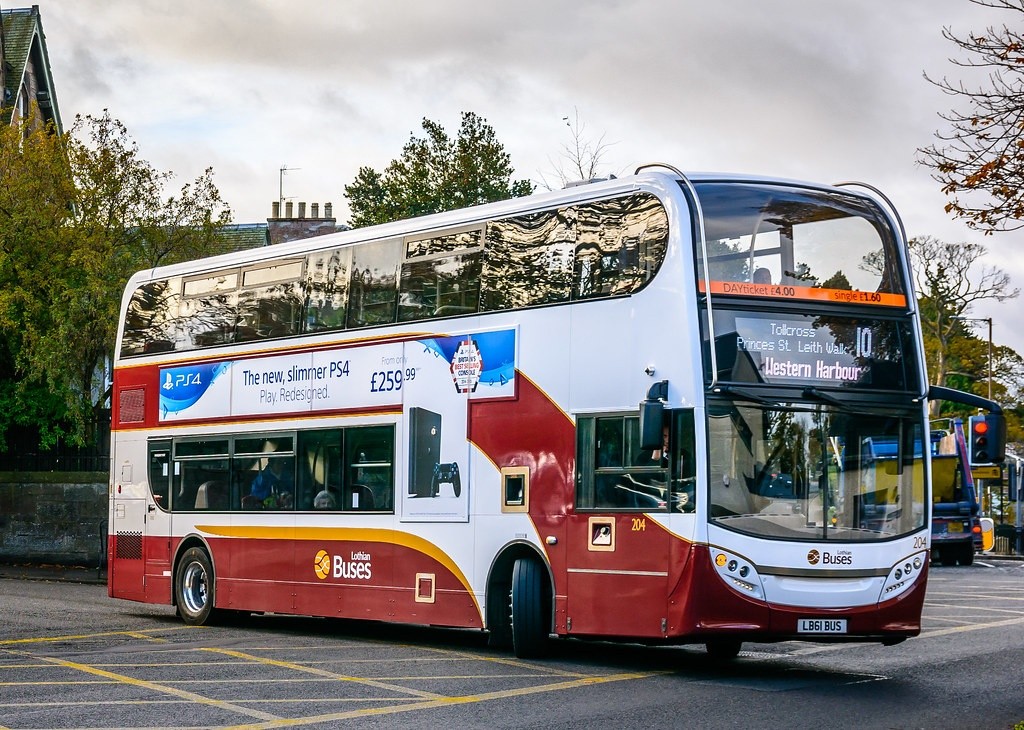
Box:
[103,159,938,667]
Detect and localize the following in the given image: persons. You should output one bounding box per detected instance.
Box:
[634,422,692,509]
[251,449,295,501]
[755,267,771,285]
[314,491,336,509]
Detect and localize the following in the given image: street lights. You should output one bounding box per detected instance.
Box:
[949,315,993,515]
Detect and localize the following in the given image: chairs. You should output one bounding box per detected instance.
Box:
[194,478,382,511]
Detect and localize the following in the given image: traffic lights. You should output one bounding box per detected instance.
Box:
[969,413,991,466]
